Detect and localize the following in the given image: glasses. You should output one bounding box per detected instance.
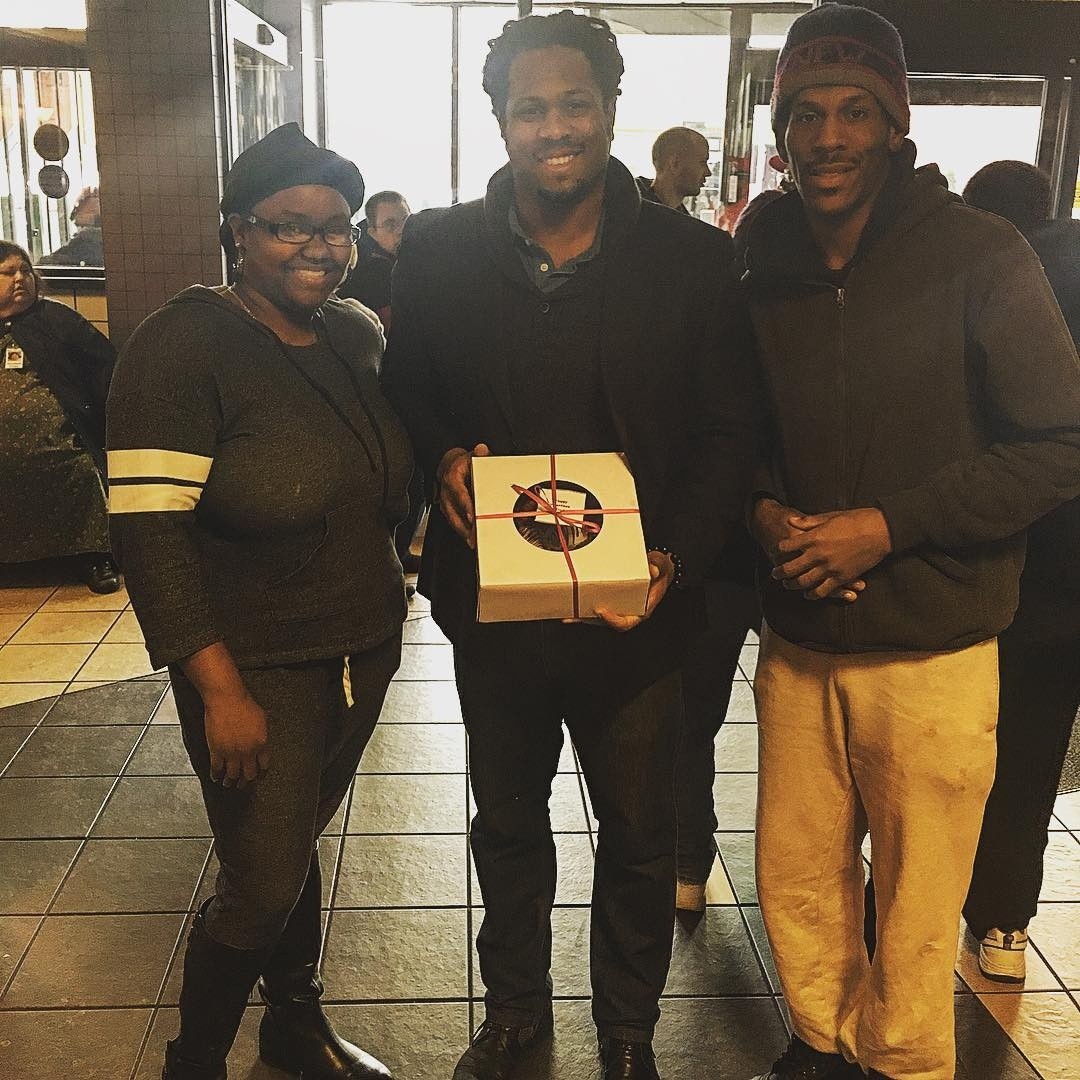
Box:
[241,211,361,247]
[372,218,407,232]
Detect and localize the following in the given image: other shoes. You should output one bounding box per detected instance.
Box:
[674,905,703,933]
[746,1034,891,1079]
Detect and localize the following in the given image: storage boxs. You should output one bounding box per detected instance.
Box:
[471,451,652,623]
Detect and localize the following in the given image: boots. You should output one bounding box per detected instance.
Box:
[258,842,392,1079]
[158,894,285,1080]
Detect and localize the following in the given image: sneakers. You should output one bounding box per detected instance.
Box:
[979,927,1028,982]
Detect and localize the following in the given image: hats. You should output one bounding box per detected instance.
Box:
[769,3,910,163]
[218,122,365,217]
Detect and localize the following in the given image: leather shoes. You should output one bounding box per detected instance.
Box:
[451,1009,552,1079]
[84,556,119,594]
[598,1030,660,1079]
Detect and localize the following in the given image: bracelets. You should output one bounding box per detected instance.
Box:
[647,547,682,587]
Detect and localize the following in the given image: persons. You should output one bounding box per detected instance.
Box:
[381,9,765,1079]
[1,125,1079,981]
[731,5,1078,1080]
[104,123,415,1079]
